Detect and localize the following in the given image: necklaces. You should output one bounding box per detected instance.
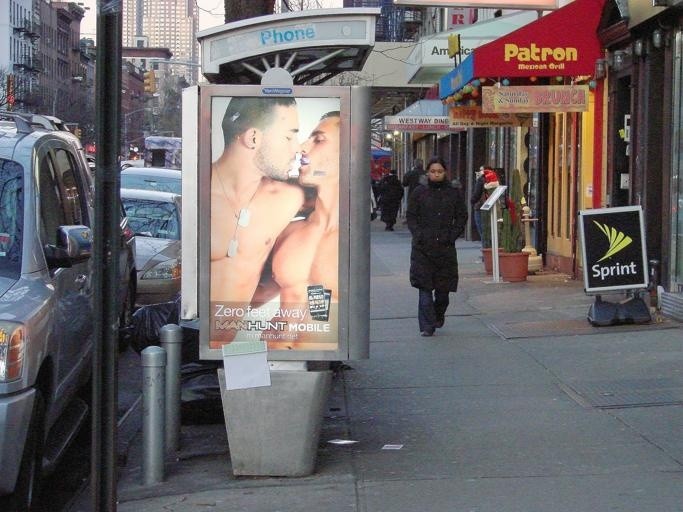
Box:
[215,164,264,258]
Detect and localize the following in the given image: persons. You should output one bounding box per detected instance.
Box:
[128,143,138,161]
[471,165,492,262]
[370,169,404,231]
[451,176,466,238]
[211,97,318,351]
[406,156,468,337]
[402,158,427,224]
[245,110,342,352]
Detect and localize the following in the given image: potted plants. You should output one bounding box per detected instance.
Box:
[481,198,504,274]
[498,169,530,282]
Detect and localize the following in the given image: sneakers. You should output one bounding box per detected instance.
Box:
[419,314,447,339]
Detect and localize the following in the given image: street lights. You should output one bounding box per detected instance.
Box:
[124,106,152,160]
[52,75,84,116]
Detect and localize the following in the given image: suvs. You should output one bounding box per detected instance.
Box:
[0,108,141,512]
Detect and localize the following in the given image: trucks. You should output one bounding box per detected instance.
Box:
[141,136,182,171]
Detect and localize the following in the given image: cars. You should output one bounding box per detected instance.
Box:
[82,151,182,305]
